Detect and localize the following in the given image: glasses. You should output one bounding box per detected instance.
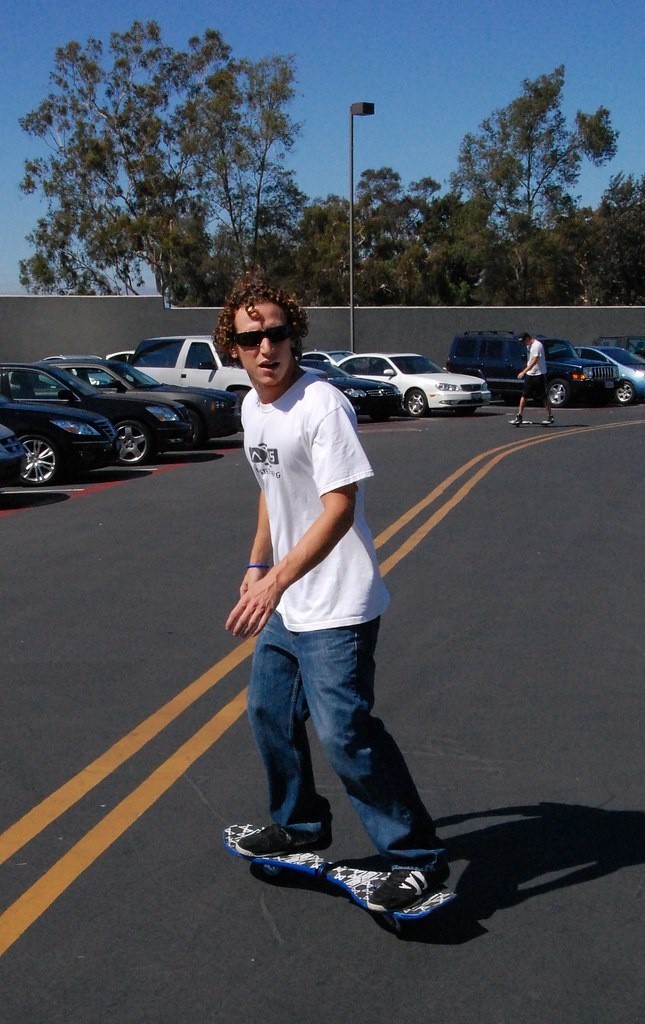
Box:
[232,323,293,348]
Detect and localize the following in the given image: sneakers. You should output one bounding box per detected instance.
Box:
[367,861,450,912]
[235,824,333,857]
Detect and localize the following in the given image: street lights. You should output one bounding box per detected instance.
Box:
[349,100,376,353]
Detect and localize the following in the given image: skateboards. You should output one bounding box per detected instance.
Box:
[221,823,459,933]
[508,420,552,428]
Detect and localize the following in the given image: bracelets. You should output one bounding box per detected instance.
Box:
[245,564,270,568]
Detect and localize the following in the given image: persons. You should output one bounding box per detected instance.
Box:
[212,272,449,915]
[513,332,555,424]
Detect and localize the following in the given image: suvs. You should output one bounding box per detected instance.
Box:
[446,329,619,408]
[124,335,328,433]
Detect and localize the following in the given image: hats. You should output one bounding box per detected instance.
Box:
[517,332,529,342]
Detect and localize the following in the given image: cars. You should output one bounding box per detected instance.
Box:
[0,425,24,482]
[8,364,220,451]
[0,363,200,467]
[299,358,402,421]
[1,394,124,487]
[547,346,645,407]
[40,349,169,371]
[33,360,240,440]
[301,348,369,373]
[327,352,491,417]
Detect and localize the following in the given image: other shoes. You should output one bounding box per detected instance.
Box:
[514,414,522,424]
[547,414,554,424]
